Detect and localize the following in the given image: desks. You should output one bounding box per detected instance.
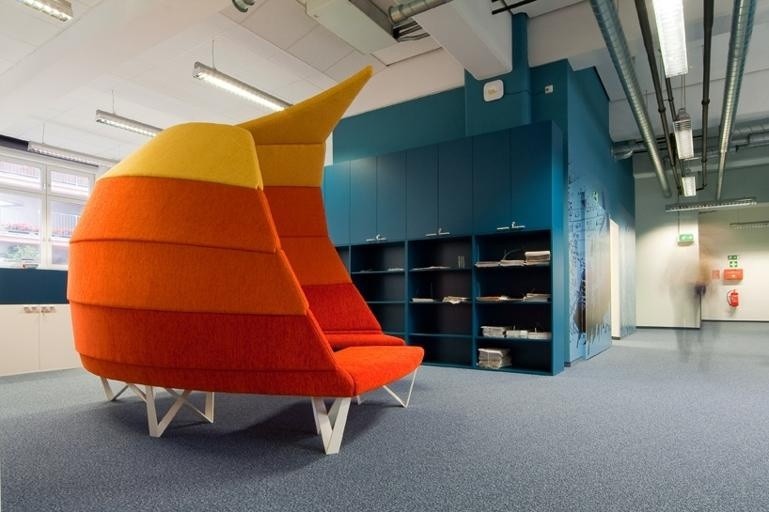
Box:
[0,268,83,377]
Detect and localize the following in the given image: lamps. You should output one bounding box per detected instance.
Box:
[652,0,696,197]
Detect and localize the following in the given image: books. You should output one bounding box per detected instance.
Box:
[411,249,551,370]
[388,267,403,271]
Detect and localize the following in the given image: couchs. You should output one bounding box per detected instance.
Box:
[67,66,424,457]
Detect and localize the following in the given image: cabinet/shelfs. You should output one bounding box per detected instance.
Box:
[406,236,475,369]
[350,150,406,245]
[472,229,564,376]
[324,162,349,247]
[472,120,564,236]
[406,136,472,240]
[350,241,407,341]
